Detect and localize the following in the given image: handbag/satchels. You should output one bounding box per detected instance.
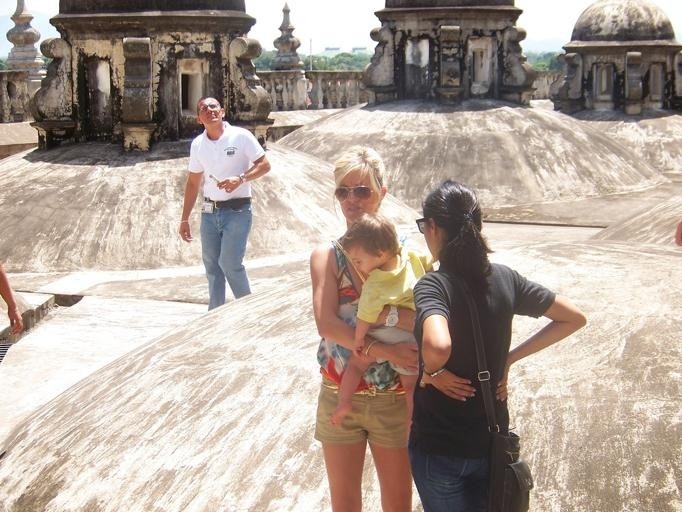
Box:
[485,429,533,512]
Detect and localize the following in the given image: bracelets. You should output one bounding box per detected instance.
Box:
[179,219,191,224]
[238,171,248,184]
[365,337,381,356]
[422,365,449,377]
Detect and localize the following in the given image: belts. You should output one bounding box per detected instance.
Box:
[203,196,252,209]
[320,375,408,397]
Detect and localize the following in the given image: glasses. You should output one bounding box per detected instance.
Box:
[415,215,434,234]
[335,186,381,200]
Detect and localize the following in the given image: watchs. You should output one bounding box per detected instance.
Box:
[385,301,401,328]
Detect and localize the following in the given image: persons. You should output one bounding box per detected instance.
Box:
[308,145,419,512]
[328,214,436,442]
[1,263,26,334]
[177,95,273,309]
[407,180,587,510]
